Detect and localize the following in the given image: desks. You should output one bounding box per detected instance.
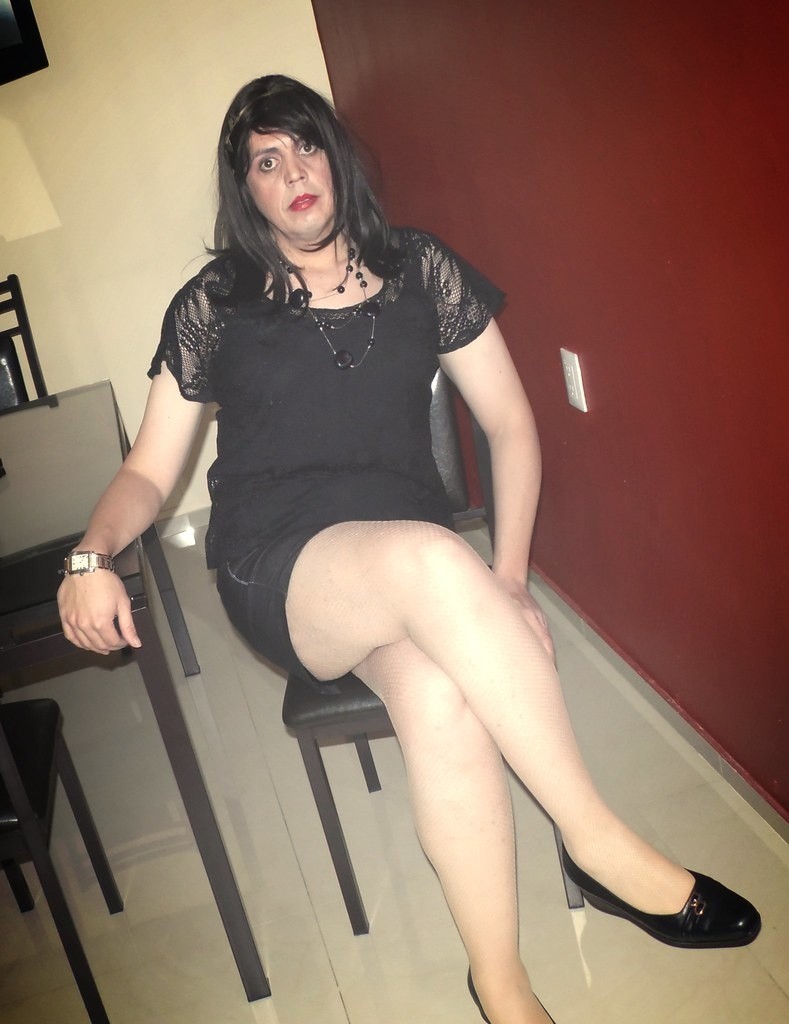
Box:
[0,380,273,1004]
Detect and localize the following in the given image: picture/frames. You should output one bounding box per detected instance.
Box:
[0,1,49,88]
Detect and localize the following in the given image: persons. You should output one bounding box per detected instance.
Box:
[58,76,763,1024]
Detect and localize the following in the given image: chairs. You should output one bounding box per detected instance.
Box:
[0,697,123,1024]
[0,274,47,410]
[280,373,583,937]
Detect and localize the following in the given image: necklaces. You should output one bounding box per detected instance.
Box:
[277,246,380,370]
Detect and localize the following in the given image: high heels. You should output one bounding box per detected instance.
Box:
[562,840,761,949]
[467,964,557,1024]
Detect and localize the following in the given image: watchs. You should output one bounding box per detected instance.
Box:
[61,550,114,577]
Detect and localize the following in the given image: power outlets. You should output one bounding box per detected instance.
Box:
[558,348,586,414]
